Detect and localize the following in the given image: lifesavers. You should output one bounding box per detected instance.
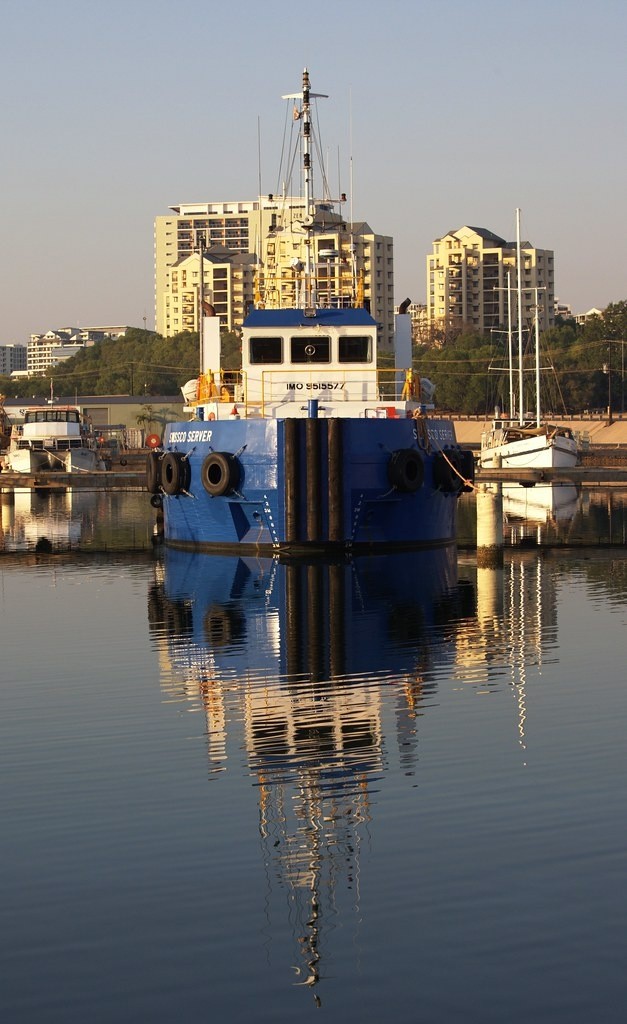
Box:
[146,451,165,494]
[201,453,240,494]
[162,453,191,495]
[387,449,424,494]
[434,450,464,492]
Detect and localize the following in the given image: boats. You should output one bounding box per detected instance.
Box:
[160,551,458,1007]
[478,207,584,469]
[146,69,459,557]
[477,474,582,755]
[3,375,106,472]
